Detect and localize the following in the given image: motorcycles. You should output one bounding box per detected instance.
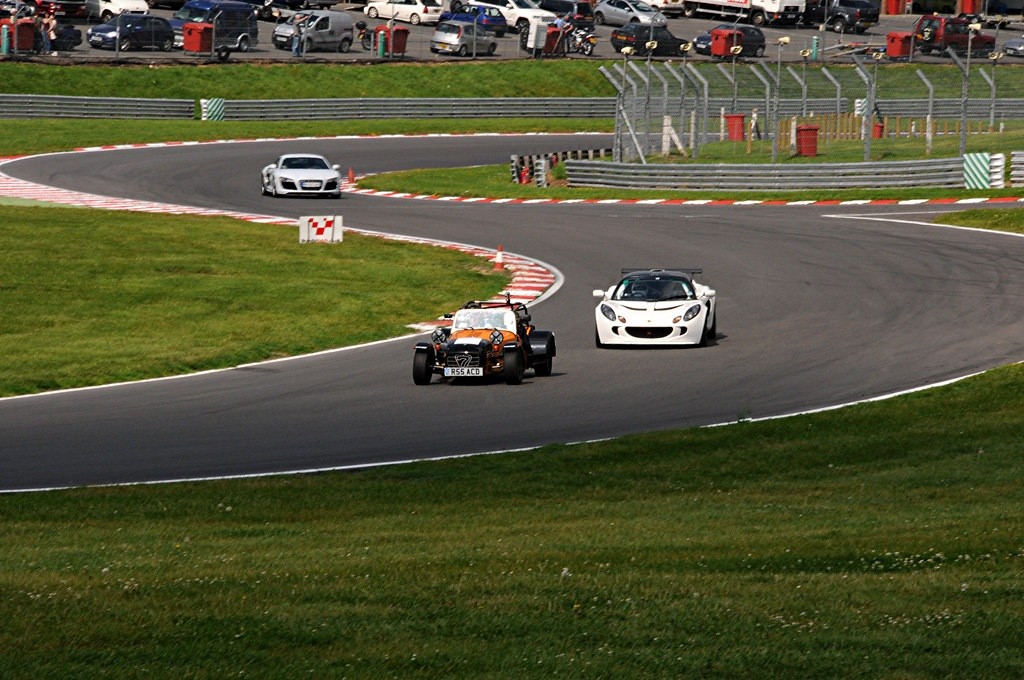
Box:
[548,14,599,56]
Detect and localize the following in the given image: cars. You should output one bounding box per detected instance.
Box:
[538,0,597,31]
[1002,32,1024,57]
[691,23,767,58]
[804,0,880,34]
[86,13,175,51]
[363,0,447,25]
[0,0,344,21]
[437,4,510,38]
[610,22,689,57]
[638,0,686,19]
[591,0,668,30]
[466,0,557,35]
[430,20,498,58]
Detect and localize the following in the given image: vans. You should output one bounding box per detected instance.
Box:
[165,0,259,51]
[271,9,355,54]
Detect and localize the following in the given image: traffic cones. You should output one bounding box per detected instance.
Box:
[521,167,531,187]
[492,242,505,272]
[348,168,357,184]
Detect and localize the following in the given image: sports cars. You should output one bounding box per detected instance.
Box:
[411,293,556,387]
[592,267,718,349]
[261,153,342,200]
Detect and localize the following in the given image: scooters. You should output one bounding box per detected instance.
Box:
[355,12,402,52]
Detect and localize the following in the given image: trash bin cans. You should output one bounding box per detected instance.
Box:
[374,25,410,58]
[543,28,566,55]
[796,124,820,157]
[0,19,36,56]
[183,23,213,56]
[725,114,746,142]
[887,32,917,61]
[873,124,884,139]
[709,30,743,60]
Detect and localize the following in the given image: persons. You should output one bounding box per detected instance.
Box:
[30,12,44,54]
[40,12,50,54]
[922,22,934,42]
[632,281,648,298]
[46,14,58,56]
[559,15,574,57]
[291,14,308,57]
[554,14,562,28]
[277,8,281,17]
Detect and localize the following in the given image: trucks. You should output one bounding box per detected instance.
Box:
[682,0,806,28]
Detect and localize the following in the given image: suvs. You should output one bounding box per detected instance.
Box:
[916,14,996,59]
